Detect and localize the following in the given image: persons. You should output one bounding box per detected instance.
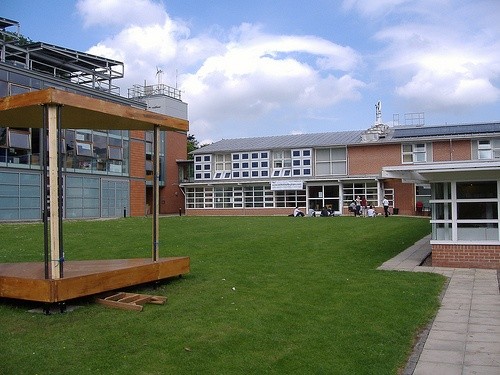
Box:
[416,200,423,215]
[382,196,390,217]
[321,206,328,216]
[356,196,360,210]
[368,206,376,217]
[362,198,367,217]
[309,208,315,217]
[294,207,305,217]
[350,200,362,215]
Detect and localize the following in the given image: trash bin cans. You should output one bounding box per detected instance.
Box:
[393,208,398,214]
